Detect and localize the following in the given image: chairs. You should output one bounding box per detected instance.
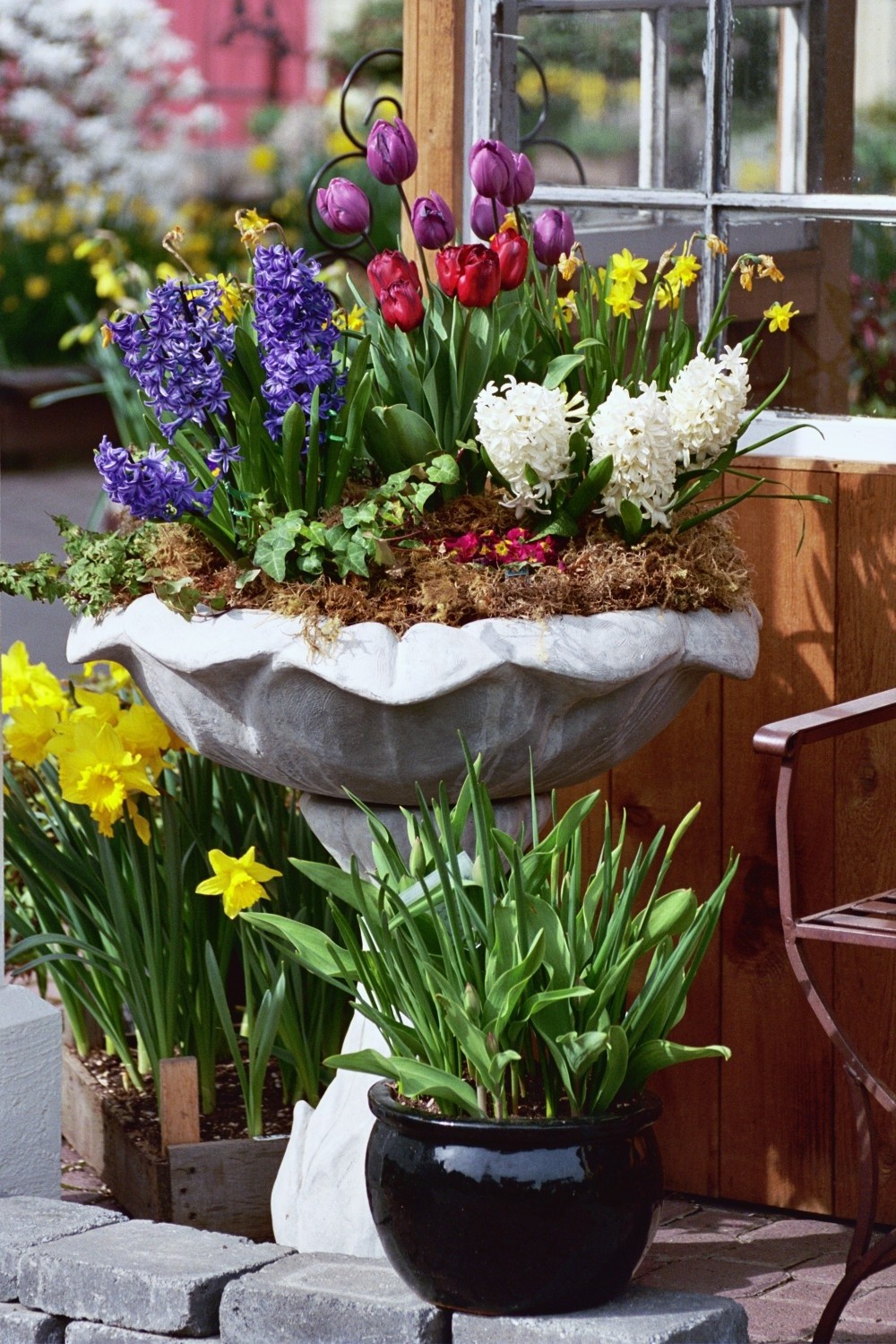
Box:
[751,689,896,1344]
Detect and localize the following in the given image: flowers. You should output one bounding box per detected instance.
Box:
[1,639,359,1139]
[0,116,835,624]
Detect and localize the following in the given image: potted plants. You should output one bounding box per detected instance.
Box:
[233,725,742,1316]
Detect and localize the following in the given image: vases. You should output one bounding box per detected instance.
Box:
[61,1032,290,1243]
[0,365,124,459]
[67,576,763,804]
